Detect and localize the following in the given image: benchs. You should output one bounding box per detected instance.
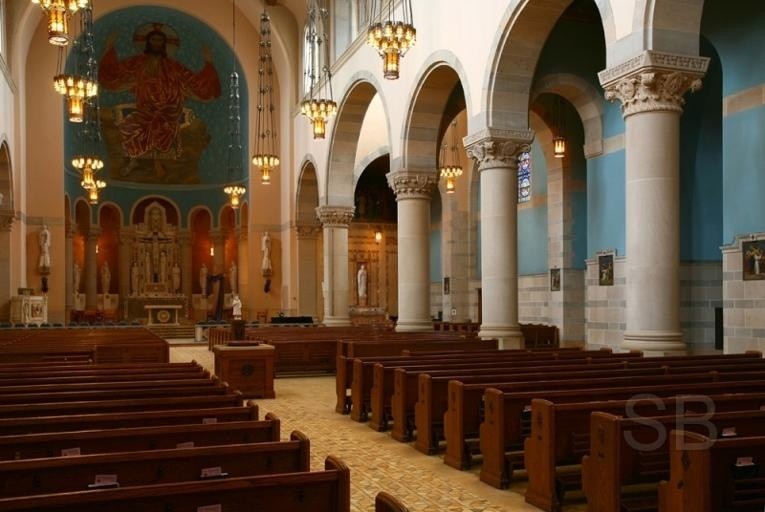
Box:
[3,327,412,512]
[208,324,737,512]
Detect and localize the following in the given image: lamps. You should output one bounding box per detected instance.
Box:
[251,2,279,186]
[367,1,418,82]
[29,0,108,205]
[299,2,338,141]
[224,3,248,209]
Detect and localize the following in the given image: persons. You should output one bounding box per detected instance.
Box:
[99,260,111,295]
[262,231,271,272]
[357,264,367,296]
[172,263,181,290]
[229,260,237,293]
[92,21,223,184]
[199,263,207,295]
[130,261,139,297]
[232,296,242,319]
[38,224,51,271]
[73,262,80,294]
